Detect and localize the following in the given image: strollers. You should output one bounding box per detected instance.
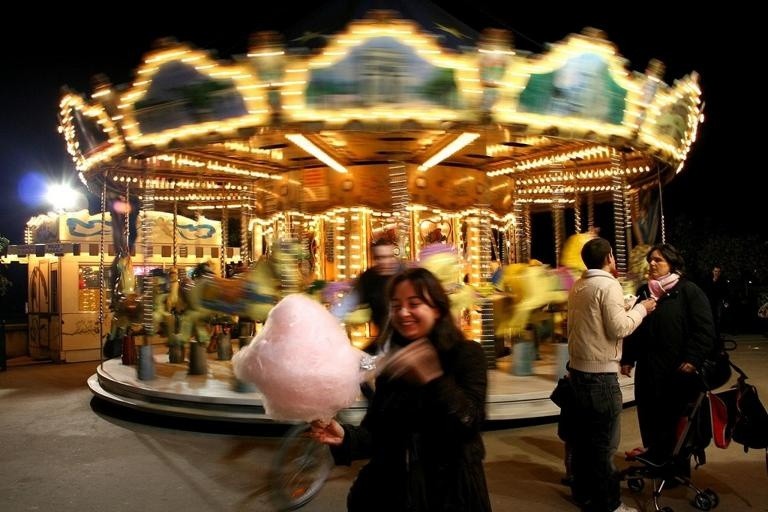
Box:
[618,363,721,512]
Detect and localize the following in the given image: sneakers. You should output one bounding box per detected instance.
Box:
[613,501,638,512]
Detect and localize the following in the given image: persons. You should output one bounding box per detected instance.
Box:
[309,269,492,512]
[698,266,730,337]
[620,244,714,490]
[329,240,414,356]
[551,239,657,512]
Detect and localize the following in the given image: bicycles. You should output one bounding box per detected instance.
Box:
[269,420,333,510]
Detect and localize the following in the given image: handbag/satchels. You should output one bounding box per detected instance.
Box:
[659,332,732,392]
